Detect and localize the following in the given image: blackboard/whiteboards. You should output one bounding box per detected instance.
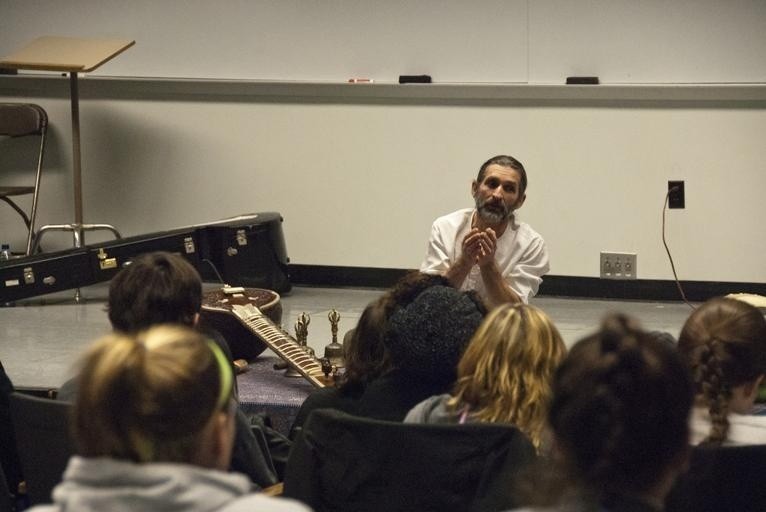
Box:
[0,0,766,109]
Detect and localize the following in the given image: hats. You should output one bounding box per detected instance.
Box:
[379,285,484,378]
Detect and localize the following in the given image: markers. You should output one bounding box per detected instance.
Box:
[347,77,374,85]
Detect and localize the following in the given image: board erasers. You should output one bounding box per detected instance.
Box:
[399,75,431,85]
[565,76,600,86]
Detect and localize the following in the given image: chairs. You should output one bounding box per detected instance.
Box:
[282,408,537,512]
[249,424,279,483]
[0,102,48,260]
[673,442,766,512]
[8,389,75,508]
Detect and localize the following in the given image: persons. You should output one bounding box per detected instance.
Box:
[18,319,316,512]
[669,294,764,452]
[401,299,572,463]
[359,283,491,422]
[287,293,396,442]
[53,248,293,483]
[412,155,551,313]
[476,312,697,509]
[388,272,455,304]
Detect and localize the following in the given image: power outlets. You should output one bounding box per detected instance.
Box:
[600,252,637,280]
[667,180,685,209]
